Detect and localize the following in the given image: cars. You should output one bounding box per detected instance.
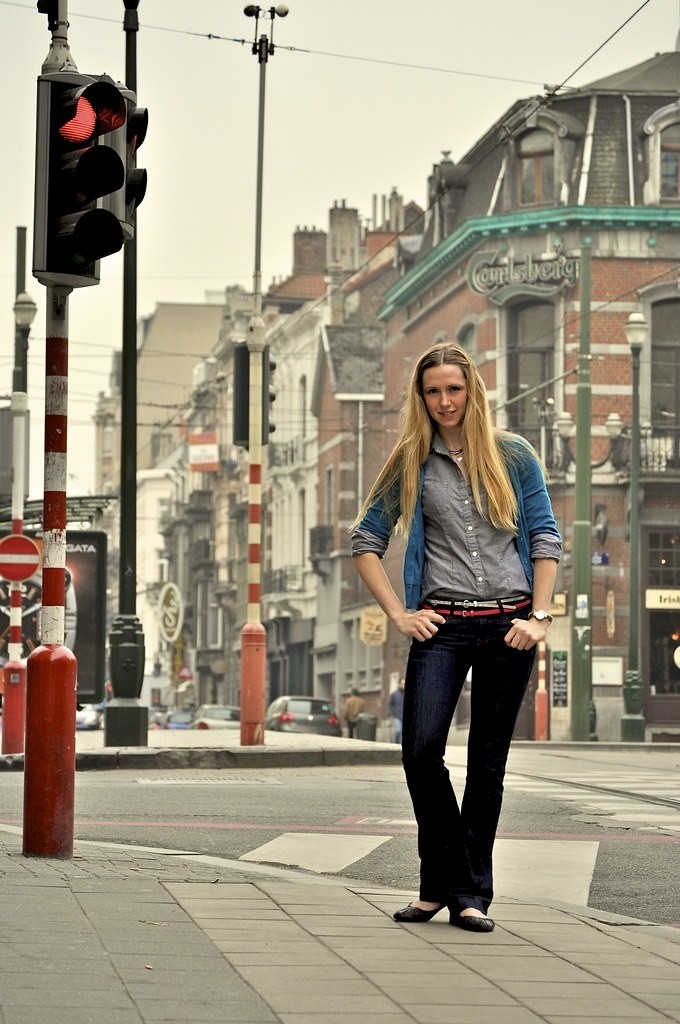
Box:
[75,706,101,731]
[191,704,242,730]
[266,697,342,738]
[149,710,192,728]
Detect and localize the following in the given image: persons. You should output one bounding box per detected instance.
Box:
[343,688,366,739]
[351,342,561,934]
[386,677,406,744]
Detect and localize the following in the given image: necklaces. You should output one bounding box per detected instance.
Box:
[449,447,466,461]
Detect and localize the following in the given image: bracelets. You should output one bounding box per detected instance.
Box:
[527,609,554,626]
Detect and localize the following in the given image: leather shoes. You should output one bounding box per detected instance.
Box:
[450,910,495,931]
[393,902,447,922]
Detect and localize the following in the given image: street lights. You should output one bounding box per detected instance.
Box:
[618,315,650,741]
[8,294,39,394]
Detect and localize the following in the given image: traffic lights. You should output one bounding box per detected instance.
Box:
[34,72,149,287]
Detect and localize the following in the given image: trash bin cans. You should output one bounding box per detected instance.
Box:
[357,714,377,741]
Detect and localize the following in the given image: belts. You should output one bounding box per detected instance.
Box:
[422,595,531,617]
